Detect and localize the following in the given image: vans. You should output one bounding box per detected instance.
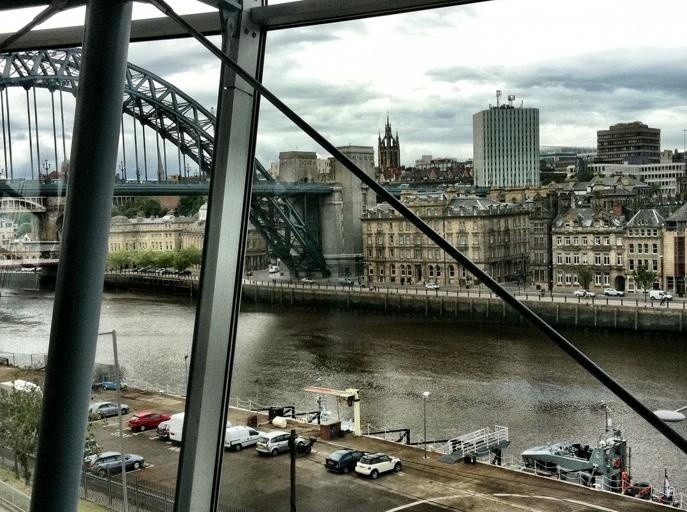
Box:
[650,291,672,301]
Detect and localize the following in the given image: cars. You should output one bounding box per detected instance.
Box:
[301,277,314,283]
[574,288,625,301]
[88,401,303,456]
[268,265,279,272]
[133,267,192,275]
[84,451,145,478]
[425,283,440,289]
[324,448,402,479]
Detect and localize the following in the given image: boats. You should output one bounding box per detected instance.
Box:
[521,407,631,491]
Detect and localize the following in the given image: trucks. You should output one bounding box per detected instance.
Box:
[338,277,354,285]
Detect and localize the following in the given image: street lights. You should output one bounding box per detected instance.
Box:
[422,391,431,459]
[288,429,317,512]
[185,355,189,384]
